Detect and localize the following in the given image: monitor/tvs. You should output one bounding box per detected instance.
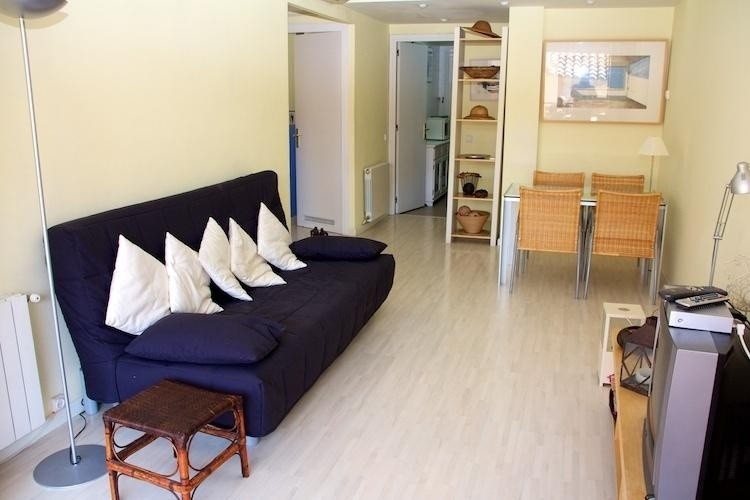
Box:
[642,304,750,500]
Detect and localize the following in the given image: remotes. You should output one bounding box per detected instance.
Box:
[659,286,728,304]
[675,293,730,310]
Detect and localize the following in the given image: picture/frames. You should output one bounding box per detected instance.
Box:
[543,41,666,124]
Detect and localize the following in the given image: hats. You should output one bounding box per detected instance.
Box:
[461,21,501,38]
[464,106,495,120]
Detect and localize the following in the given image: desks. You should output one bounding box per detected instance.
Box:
[613,326,653,499]
[498,184,668,300]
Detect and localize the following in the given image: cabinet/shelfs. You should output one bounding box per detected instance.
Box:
[426,141,448,207]
[598,303,646,387]
[445,25,508,247]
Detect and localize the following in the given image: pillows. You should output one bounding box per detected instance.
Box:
[288,237,387,260]
[228,217,287,287]
[126,314,289,364]
[256,201,308,270]
[197,218,252,300]
[105,234,171,335]
[164,231,225,314]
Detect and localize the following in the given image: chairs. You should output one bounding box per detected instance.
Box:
[591,173,644,197]
[583,190,660,305]
[533,171,585,200]
[508,185,580,298]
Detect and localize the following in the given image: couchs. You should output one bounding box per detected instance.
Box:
[47,170,395,446]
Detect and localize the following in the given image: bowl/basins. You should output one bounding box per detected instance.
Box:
[454,211,490,234]
[635,368,652,385]
[459,66,500,79]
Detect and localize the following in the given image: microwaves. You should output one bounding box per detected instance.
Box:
[425,117,450,141]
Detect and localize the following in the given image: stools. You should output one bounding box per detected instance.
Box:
[102,381,250,500]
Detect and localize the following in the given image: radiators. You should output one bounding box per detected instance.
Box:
[0,292,48,453]
[362,161,390,225]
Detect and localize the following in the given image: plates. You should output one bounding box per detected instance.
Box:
[458,153,490,160]
[616,325,658,355]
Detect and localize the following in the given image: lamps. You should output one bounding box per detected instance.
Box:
[638,136,669,193]
[709,162,750,290]
